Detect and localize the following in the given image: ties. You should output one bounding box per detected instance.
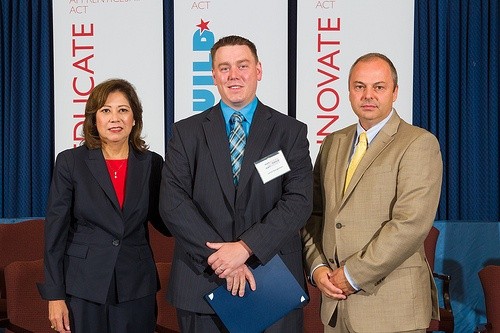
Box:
[229,114,246,185]
[344,133,368,193]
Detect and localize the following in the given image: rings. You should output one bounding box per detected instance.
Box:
[51,326,54,328]
[219,267,224,271]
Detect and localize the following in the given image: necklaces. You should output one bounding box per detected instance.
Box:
[109,156,126,178]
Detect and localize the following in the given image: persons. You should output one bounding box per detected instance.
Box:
[36,80,174,333]
[300,53,442,333]
[159,35,314,333]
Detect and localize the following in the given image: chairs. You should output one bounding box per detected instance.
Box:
[0,219,500,333]
